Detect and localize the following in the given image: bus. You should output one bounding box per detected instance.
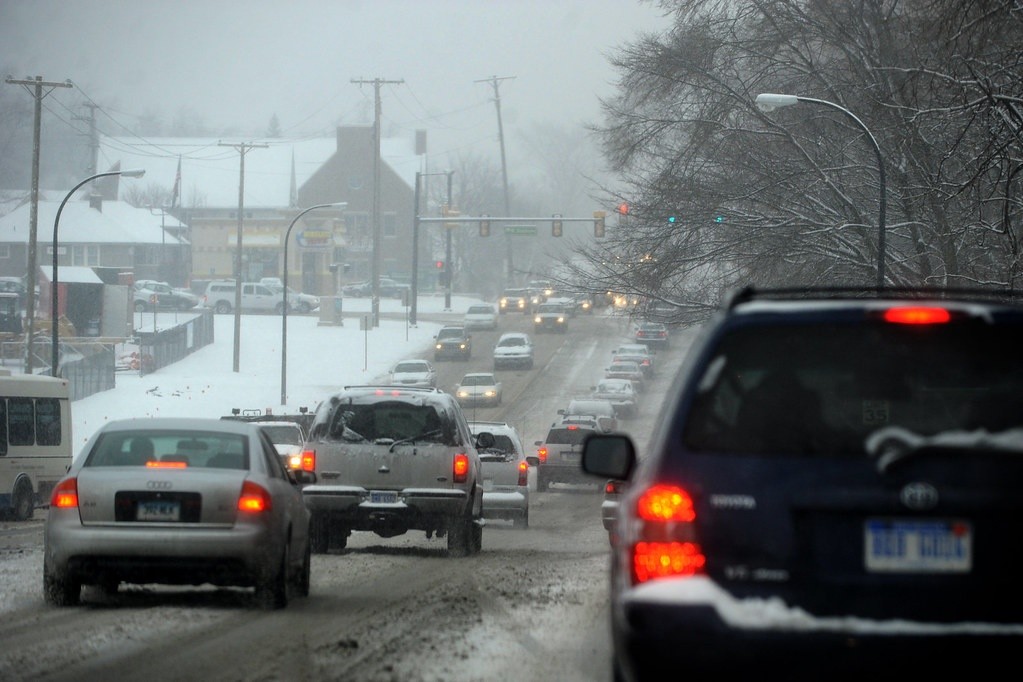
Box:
[0,373,73,522]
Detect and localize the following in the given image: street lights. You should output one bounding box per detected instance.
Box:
[757,93,885,297]
[281,201,347,405]
[52,169,146,378]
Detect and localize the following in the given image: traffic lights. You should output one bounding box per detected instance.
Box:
[667,205,675,222]
[620,203,628,228]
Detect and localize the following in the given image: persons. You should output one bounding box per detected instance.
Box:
[352,407,377,426]
[129,436,156,456]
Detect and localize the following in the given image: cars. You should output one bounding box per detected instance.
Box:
[133,279,198,313]
[455,373,501,408]
[343,274,409,300]
[558,305,679,432]
[467,280,579,370]
[43,418,318,614]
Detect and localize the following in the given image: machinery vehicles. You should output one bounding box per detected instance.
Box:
[0,292,76,367]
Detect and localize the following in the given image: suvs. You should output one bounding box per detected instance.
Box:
[433,324,472,361]
[292,383,540,558]
[534,414,609,492]
[580,283,1023,682]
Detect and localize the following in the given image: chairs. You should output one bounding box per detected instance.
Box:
[110,452,190,466]
[353,408,376,435]
[205,452,246,470]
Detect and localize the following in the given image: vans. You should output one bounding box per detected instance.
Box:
[203,280,293,315]
[389,360,436,388]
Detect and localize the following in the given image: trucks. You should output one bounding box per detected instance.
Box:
[222,407,315,477]
